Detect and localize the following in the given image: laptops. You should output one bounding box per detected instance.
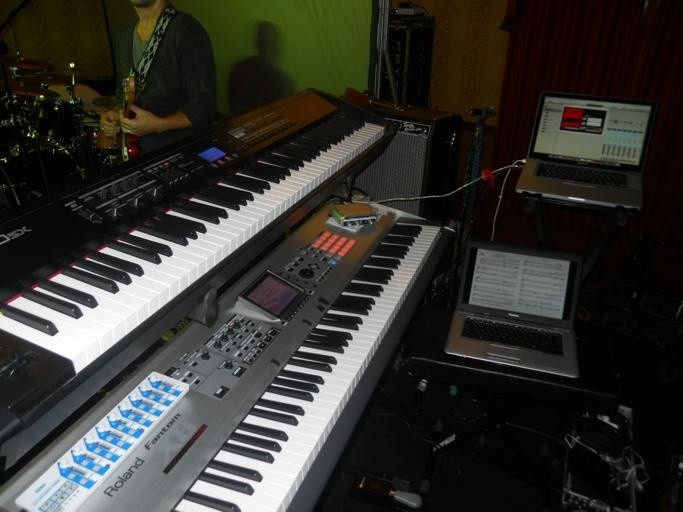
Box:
[444,240,585,378]
[515,90,659,211]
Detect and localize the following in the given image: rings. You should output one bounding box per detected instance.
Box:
[127,130,130,134]
[101,128,105,132]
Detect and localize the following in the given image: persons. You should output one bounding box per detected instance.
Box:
[100,0,217,156]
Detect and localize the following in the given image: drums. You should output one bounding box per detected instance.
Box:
[25,96,83,145]
[0,94,49,156]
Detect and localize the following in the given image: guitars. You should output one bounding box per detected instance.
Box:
[121,70,136,162]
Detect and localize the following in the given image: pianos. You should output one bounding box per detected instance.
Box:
[1,88,457,511]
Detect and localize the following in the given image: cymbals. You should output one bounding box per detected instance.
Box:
[47,83,108,114]
[0,56,54,69]
[0,82,60,97]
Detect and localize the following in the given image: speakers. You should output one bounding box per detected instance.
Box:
[335,105,462,218]
